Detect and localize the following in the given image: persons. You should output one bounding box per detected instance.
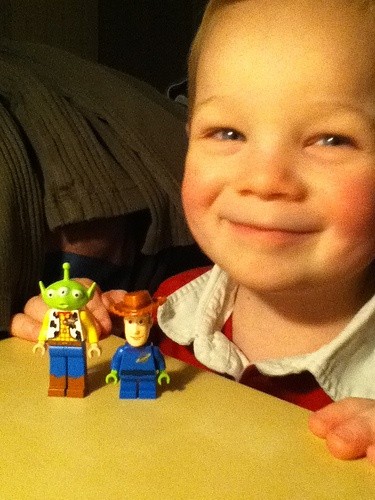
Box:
[7,1,374,467]
[1,19,212,343]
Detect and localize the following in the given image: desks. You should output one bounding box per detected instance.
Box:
[1,333,373,500]
